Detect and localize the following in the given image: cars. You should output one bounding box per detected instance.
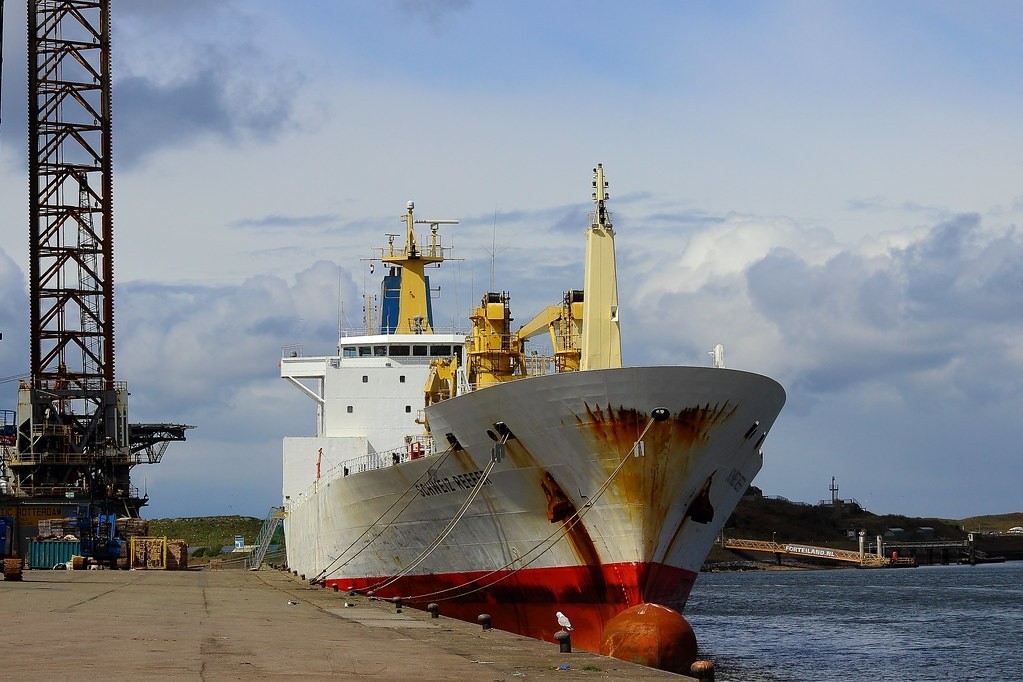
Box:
[1006,526,1023,534]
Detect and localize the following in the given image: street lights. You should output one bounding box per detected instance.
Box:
[772,531,777,553]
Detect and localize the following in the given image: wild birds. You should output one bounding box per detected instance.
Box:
[345,602,357,608]
[556,611,573,632]
[287,599,299,606]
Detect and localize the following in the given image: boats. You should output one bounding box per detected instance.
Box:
[277,159,788,649]
